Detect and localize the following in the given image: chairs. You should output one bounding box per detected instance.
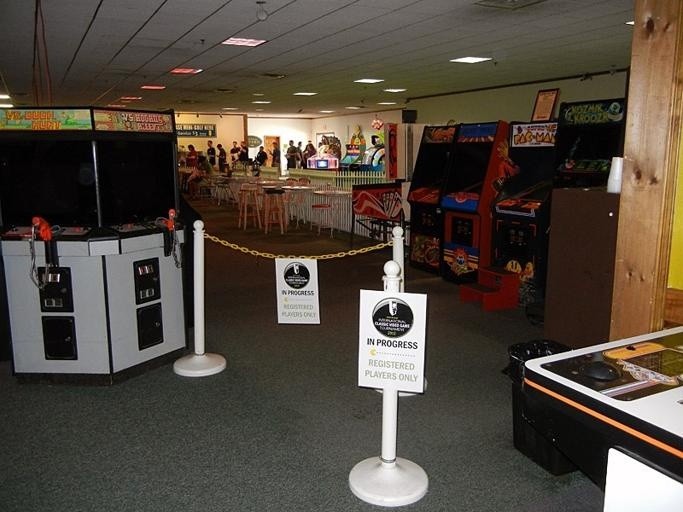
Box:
[199,176,334,239]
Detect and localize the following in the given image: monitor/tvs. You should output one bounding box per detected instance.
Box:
[447,141,493,195]
[503,155,554,201]
[565,127,623,159]
[413,142,453,190]
[0,137,98,227]
[95,139,176,229]
[316,160,328,168]
[342,155,359,164]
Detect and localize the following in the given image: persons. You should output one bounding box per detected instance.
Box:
[177,135,328,202]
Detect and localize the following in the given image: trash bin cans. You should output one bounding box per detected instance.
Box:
[500,336,580,475]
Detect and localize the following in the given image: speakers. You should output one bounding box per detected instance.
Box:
[401,109,417,123]
